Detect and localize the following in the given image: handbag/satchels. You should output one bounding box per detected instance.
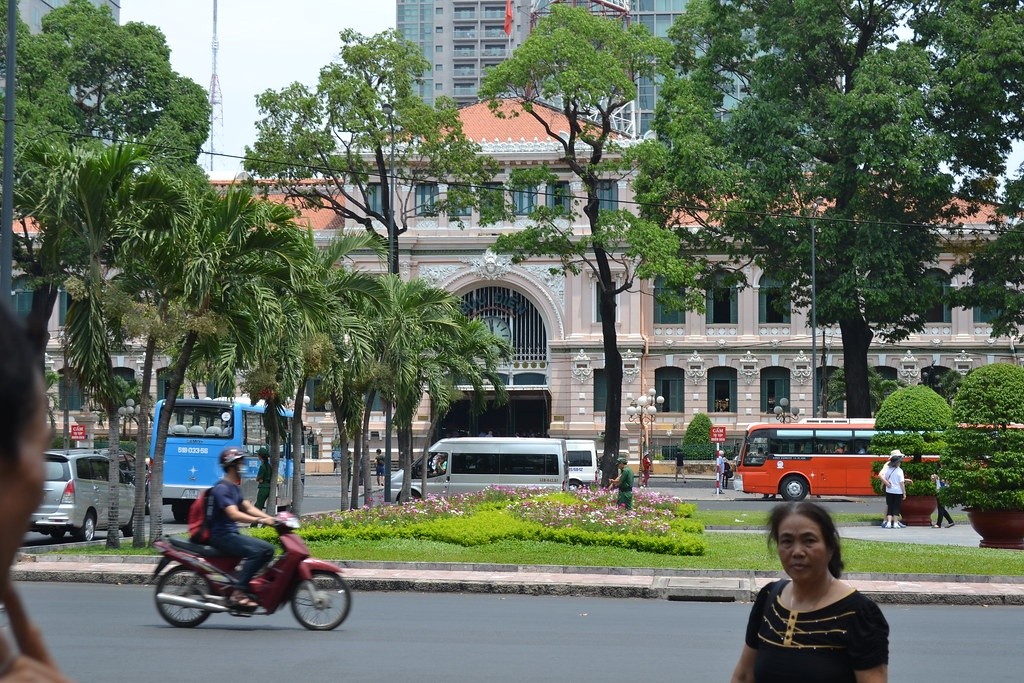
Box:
[727,471,733,478]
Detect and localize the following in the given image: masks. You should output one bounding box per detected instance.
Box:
[236,464,248,473]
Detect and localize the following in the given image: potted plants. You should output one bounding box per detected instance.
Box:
[937,363,1024,549]
[870,384,953,527]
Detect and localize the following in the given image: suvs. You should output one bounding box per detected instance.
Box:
[25,450,153,541]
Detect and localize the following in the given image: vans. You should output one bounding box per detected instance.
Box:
[565,440,598,490]
[381,437,569,507]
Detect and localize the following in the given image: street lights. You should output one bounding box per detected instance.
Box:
[627,388,665,487]
[809,196,826,418]
[118,398,140,441]
[774,397,800,424]
[382,104,393,503]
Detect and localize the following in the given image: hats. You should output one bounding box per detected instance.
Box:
[888,456,902,466]
[734,456,739,460]
[615,458,626,466]
[255,448,269,458]
[723,457,728,462]
[890,450,904,457]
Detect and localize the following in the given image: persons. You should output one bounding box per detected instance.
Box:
[433,454,447,475]
[719,450,731,494]
[0,313,74,683]
[858,445,865,453]
[930,474,955,528]
[641,453,649,487]
[762,494,776,499]
[878,450,912,529]
[731,501,889,683]
[833,443,843,453]
[347,451,353,492]
[607,457,634,510]
[250,447,272,528]
[374,449,385,486]
[674,448,686,483]
[204,449,275,614]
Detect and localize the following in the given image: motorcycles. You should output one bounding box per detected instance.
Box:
[149,512,351,631]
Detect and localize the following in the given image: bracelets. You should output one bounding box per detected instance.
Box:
[255,517,261,522]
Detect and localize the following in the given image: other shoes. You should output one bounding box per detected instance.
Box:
[945,523,954,528]
[893,523,900,528]
[885,522,891,529]
[882,522,887,528]
[891,521,906,528]
[932,525,941,528]
[224,594,258,608]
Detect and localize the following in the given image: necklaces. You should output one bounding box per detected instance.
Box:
[791,577,834,612]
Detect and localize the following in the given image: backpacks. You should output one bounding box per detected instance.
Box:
[189,482,232,544]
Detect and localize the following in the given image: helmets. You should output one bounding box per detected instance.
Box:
[220,448,244,468]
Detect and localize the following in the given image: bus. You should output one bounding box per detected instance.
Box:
[147,398,316,524]
[732,420,1024,503]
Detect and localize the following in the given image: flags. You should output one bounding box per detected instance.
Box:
[504,0,512,36]
